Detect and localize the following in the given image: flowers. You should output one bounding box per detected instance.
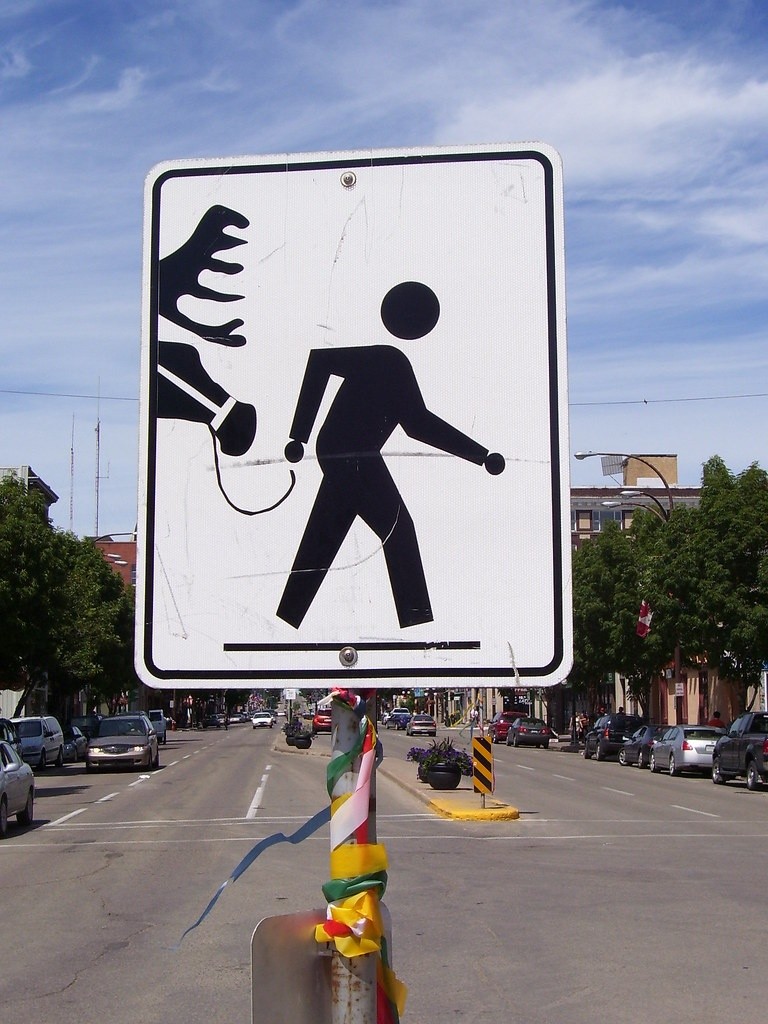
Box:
[281,717,319,740]
[407,736,472,780]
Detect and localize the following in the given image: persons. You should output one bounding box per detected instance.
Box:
[128,722,141,733]
[178,710,183,720]
[708,711,725,728]
[617,707,626,715]
[224,710,229,730]
[569,707,605,743]
[450,713,455,727]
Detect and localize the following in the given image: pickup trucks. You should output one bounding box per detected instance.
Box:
[712,711,768,790]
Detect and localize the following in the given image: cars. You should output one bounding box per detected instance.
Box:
[311,708,331,733]
[618,723,672,768]
[250,712,273,729]
[405,714,436,736]
[382,707,412,730]
[648,725,728,776]
[206,709,278,729]
[0,717,23,758]
[63,709,167,762]
[0,740,35,837]
[505,717,550,749]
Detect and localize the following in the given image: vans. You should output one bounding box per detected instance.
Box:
[9,716,64,770]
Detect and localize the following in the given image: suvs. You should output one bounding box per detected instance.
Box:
[85,714,159,772]
[585,714,641,761]
[488,711,528,743]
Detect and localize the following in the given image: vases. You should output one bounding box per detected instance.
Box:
[413,754,417,761]
[294,735,312,749]
[418,767,427,783]
[285,735,295,747]
[427,763,462,789]
